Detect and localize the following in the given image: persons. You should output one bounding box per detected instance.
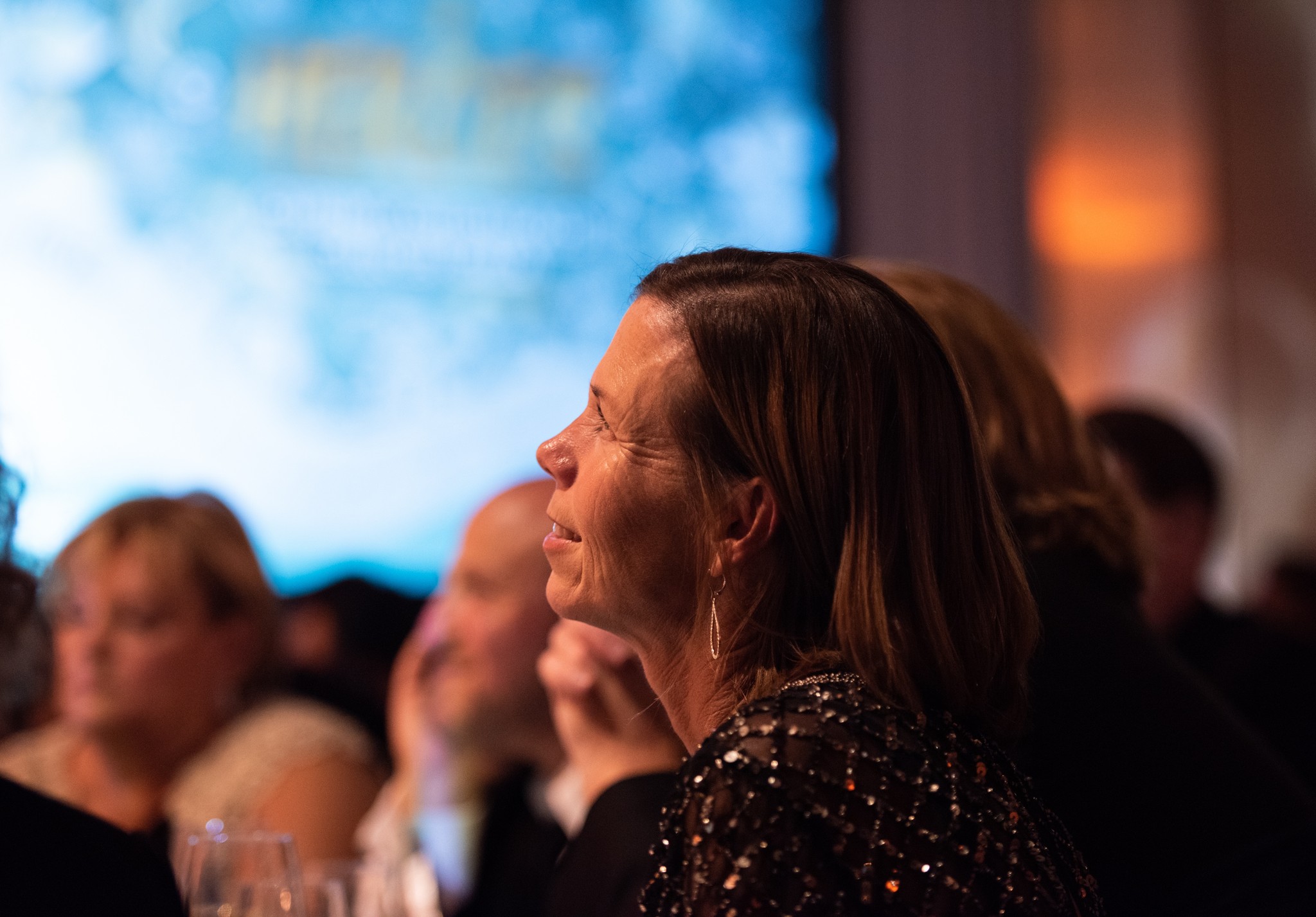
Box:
[1,248,1315,917]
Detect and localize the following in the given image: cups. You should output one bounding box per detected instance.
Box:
[178,834,309,917]
[327,852,442,917]
